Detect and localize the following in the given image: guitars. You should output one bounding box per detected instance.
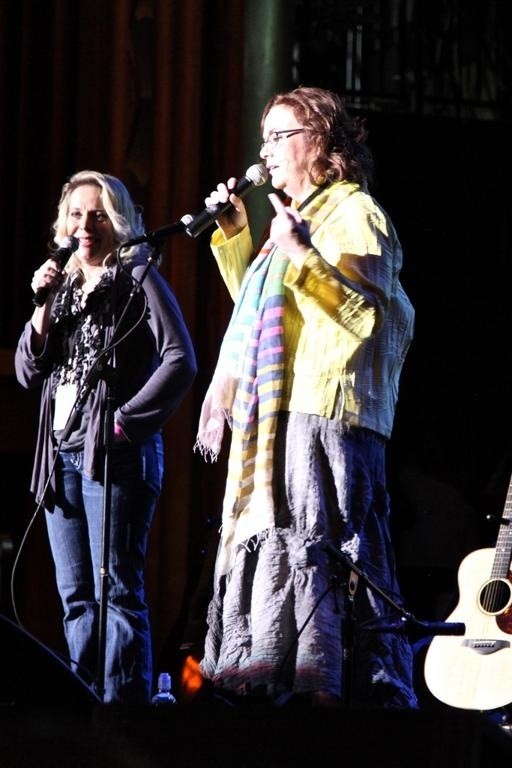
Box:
[425,472,512,710]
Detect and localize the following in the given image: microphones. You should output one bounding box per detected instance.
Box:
[365,615,466,637]
[33,235,79,308]
[124,214,194,249]
[185,163,268,239]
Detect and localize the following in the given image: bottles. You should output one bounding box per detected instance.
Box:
[149,673,176,706]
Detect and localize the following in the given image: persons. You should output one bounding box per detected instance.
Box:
[198,86,422,708]
[11,165,202,714]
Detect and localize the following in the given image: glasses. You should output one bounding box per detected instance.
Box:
[259,126,302,150]
